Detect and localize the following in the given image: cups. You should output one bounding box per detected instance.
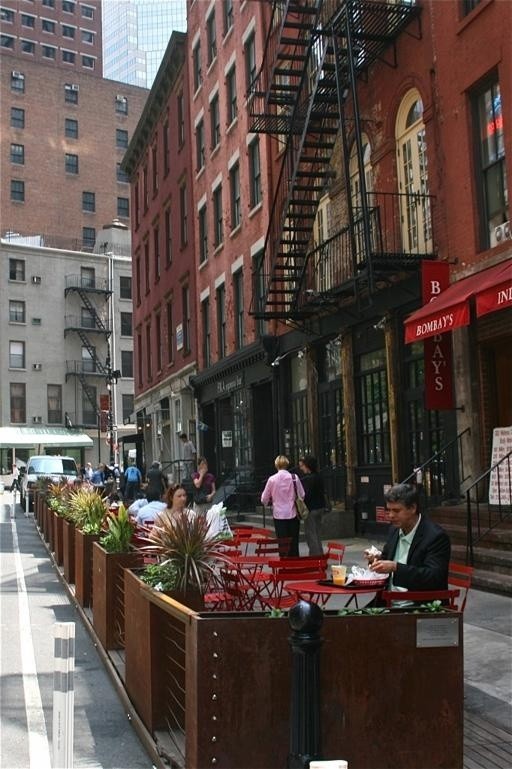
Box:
[331,564,346,585]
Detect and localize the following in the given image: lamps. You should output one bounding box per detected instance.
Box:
[329,324,346,347]
[372,313,388,332]
[271,345,307,366]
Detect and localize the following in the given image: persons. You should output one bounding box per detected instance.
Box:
[192,454,219,504]
[261,454,306,562]
[299,454,328,556]
[148,485,201,550]
[179,432,197,481]
[363,483,450,613]
[75,457,170,525]
[9,463,22,494]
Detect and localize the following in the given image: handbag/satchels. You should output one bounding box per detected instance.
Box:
[295,497,309,520]
[194,490,211,504]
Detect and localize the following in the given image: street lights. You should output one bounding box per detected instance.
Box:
[103,358,118,467]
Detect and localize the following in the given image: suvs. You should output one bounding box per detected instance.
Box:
[19,454,86,514]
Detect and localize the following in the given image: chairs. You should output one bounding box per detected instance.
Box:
[204,523,473,613]
